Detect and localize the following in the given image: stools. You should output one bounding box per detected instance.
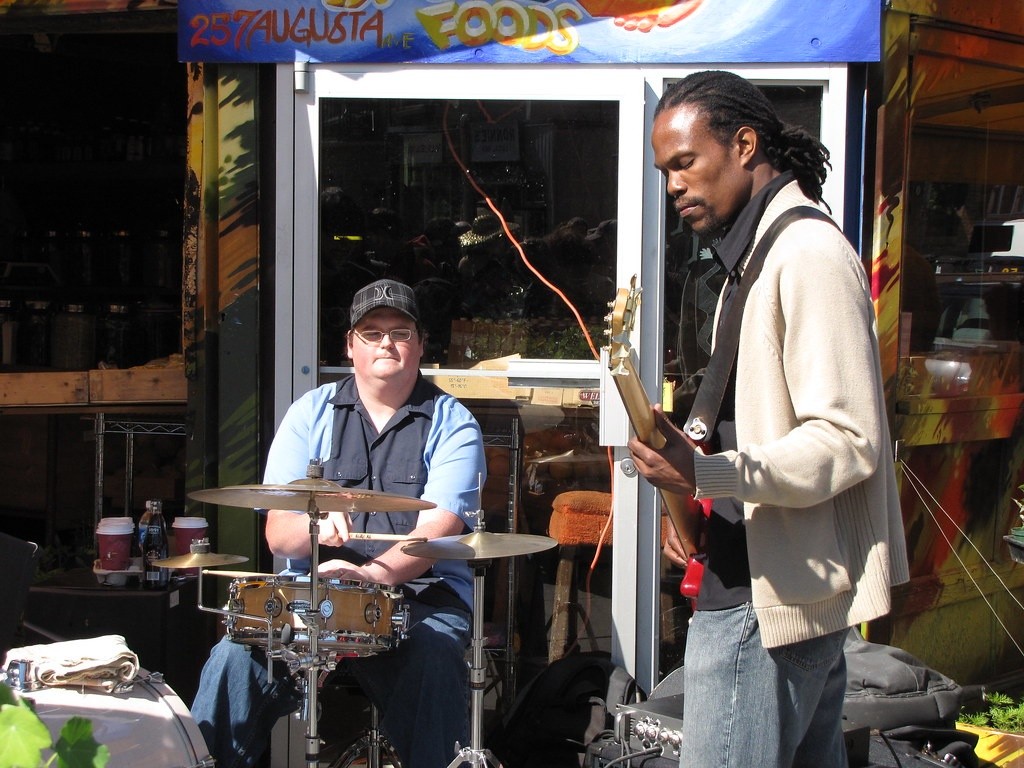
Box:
[549,491,689,661]
[323,676,404,768]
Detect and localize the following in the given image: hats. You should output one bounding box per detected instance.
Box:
[349,279,419,328]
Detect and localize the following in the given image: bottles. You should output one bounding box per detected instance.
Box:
[138,501,166,582]
[142,499,170,593]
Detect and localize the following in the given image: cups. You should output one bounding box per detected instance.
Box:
[123,517,135,528]
[96,518,133,570]
[172,516,209,576]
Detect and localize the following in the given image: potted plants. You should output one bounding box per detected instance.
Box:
[956,691,1024,768]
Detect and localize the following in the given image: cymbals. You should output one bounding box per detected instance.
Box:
[149,549,253,572]
[398,527,562,564]
[181,473,442,519]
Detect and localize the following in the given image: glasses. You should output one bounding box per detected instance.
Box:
[353,328,419,344]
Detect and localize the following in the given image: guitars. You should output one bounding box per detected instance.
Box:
[595,268,721,620]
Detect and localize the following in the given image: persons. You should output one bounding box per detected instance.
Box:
[313,186,626,363]
[901,185,991,355]
[186,279,488,768]
[626,68,909,768]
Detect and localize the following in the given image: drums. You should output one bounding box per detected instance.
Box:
[217,568,416,660]
[0,656,221,768]
